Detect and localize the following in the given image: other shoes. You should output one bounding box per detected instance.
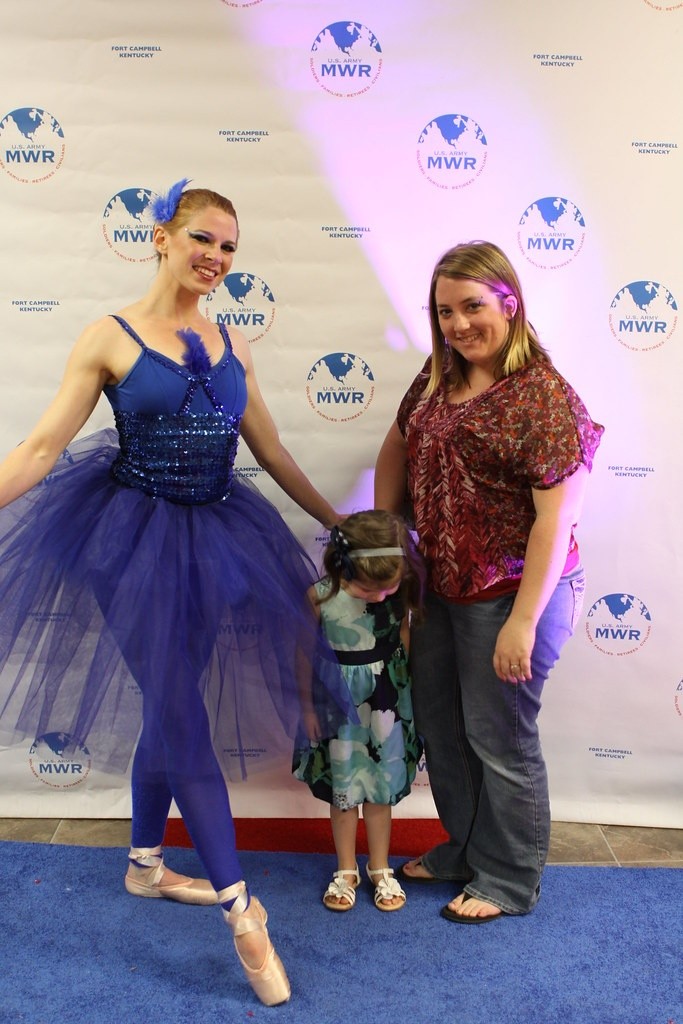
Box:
[124,846,219,905]
[218,881,291,1007]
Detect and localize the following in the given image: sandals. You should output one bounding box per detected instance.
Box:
[323,861,363,912]
[366,861,407,911]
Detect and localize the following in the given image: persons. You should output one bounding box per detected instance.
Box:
[1,178,352,1008]
[297,510,426,911]
[374,241,604,924]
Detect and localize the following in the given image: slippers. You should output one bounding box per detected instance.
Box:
[398,853,445,885]
[440,890,502,923]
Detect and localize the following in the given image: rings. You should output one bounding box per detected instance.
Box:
[510,664,520,670]
